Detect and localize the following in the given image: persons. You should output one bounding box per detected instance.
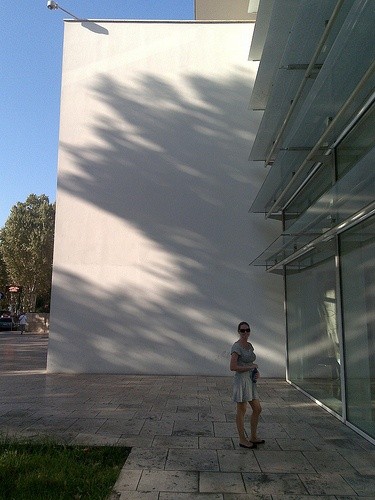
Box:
[13,312,27,335]
[229,322,266,450]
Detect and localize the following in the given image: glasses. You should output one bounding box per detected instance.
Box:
[239,328,250,333]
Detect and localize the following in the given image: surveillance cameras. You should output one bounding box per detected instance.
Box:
[47,0,54,10]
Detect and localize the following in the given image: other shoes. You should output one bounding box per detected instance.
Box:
[249,440,265,444]
[239,443,257,449]
